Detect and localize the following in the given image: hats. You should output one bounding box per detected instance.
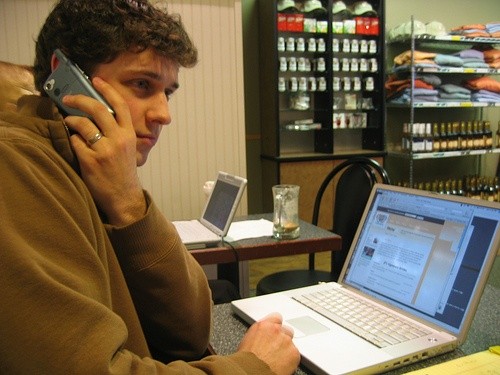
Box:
[277,0,377,15]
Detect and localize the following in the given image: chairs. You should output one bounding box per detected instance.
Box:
[255,155,391,296]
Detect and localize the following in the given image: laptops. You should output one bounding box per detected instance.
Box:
[174,171,250,245]
[229,184,500,375]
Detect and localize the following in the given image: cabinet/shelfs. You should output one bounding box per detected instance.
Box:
[384,15,500,189]
[256,0,384,230]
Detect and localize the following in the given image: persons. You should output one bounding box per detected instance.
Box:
[0,0,300,375]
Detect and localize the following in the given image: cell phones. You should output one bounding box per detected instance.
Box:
[45,50,115,125]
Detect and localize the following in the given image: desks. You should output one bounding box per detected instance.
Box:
[208,284,500,375]
[185,213,341,298]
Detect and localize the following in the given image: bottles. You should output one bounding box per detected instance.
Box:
[401,119,500,154]
[392,176,500,204]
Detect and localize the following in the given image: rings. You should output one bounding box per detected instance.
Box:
[87,133,102,145]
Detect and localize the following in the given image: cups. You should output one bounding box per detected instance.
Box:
[271,184,301,240]
